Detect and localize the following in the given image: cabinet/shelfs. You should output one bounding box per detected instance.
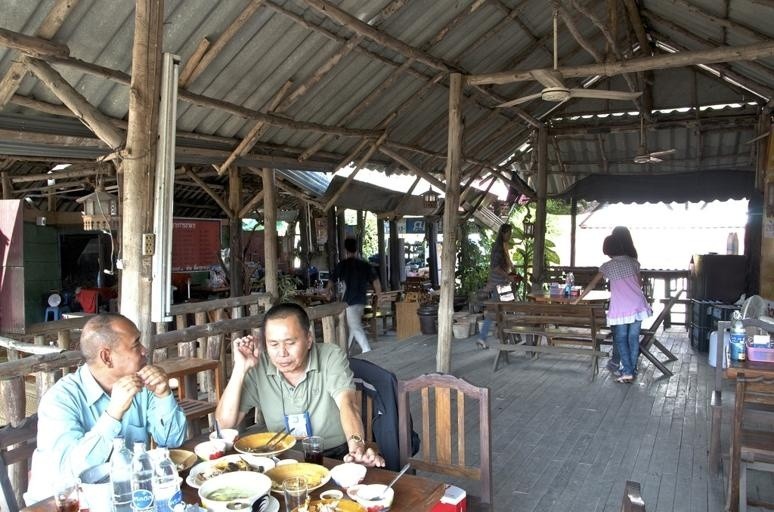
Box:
[147,358,224,449]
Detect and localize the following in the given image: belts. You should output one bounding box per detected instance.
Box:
[323,443,348,457]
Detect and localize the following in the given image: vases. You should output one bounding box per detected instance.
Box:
[452,323,469,339]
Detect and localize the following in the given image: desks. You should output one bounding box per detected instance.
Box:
[196,286,232,292]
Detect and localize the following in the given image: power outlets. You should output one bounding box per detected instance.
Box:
[142,233,156,256]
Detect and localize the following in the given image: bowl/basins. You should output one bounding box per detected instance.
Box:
[198,472,272,512]
[209,429,239,451]
[264,464,331,496]
[168,450,197,472]
[234,432,297,455]
[195,441,227,462]
[356,483,394,512]
[331,463,367,488]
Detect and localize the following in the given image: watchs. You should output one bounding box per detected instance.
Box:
[347,433,366,446]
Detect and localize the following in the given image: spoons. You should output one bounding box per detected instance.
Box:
[212,416,223,439]
[369,462,412,501]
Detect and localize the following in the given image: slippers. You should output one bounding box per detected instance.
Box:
[613,377,632,385]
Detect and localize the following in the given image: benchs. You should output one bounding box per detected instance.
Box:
[484,300,608,382]
[493,280,537,354]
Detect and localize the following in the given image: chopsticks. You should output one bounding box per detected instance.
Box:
[265,427,296,448]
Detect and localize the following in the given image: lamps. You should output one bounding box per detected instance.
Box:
[152,51,181,325]
[553,8,559,71]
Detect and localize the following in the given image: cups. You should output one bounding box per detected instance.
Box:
[302,436,324,466]
[55,472,80,512]
[225,503,252,512]
[283,476,308,512]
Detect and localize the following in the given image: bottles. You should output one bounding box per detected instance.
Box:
[733,231,739,255]
[570,272,574,288]
[728,309,747,362]
[727,233,733,254]
[130,441,154,512]
[565,273,570,296]
[110,435,134,506]
[313,280,319,295]
[320,280,324,294]
[151,447,181,512]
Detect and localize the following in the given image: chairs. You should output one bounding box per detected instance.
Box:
[343,380,376,461]
[711,321,731,474]
[0,413,40,511]
[398,374,491,512]
[362,295,378,344]
[724,373,773,510]
[639,288,685,376]
[622,479,645,510]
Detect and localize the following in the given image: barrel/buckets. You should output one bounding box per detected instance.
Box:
[416,305,438,336]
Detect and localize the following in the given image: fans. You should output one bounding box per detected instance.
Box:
[630,113,678,165]
[493,70,642,110]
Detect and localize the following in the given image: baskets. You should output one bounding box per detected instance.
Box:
[745,340,774,362]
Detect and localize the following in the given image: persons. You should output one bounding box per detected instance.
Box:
[475,223,516,350]
[325,237,382,354]
[608,225,637,368]
[23,313,187,505]
[77,239,102,313]
[569,235,654,382]
[215,303,386,469]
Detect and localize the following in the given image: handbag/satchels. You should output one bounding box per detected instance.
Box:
[496,282,515,302]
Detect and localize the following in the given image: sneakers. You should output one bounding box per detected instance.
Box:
[606,359,620,370]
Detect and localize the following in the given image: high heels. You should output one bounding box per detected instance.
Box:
[476,339,489,350]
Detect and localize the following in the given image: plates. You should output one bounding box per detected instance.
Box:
[78,462,118,486]
[264,496,281,512]
[186,454,275,489]
[277,458,298,465]
[347,484,367,501]
[180,503,206,512]
[320,490,343,500]
[290,499,369,512]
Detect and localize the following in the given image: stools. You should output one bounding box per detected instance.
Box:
[660,298,689,330]
[44,307,68,323]
